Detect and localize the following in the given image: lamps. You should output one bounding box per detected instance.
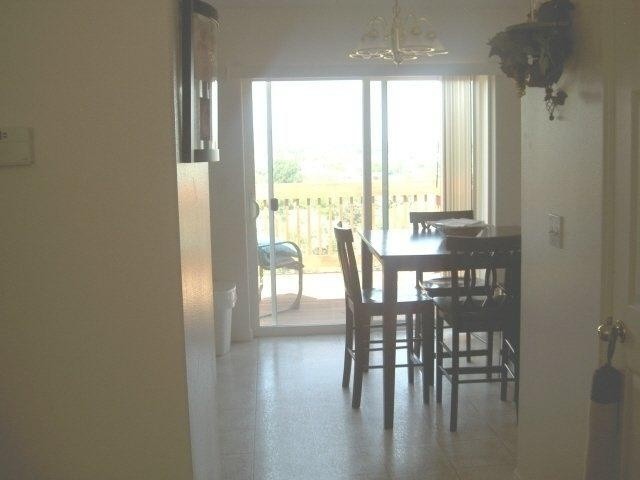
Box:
[344,1,448,67]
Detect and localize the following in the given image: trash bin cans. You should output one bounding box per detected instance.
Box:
[214,282,237,356]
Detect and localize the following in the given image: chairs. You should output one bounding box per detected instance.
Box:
[433,233,521,431]
[334,220,435,409]
[255,201,304,320]
[409,211,494,380]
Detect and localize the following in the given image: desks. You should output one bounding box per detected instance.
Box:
[355,226,520,431]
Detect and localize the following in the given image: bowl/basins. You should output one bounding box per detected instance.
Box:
[426,220,488,239]
